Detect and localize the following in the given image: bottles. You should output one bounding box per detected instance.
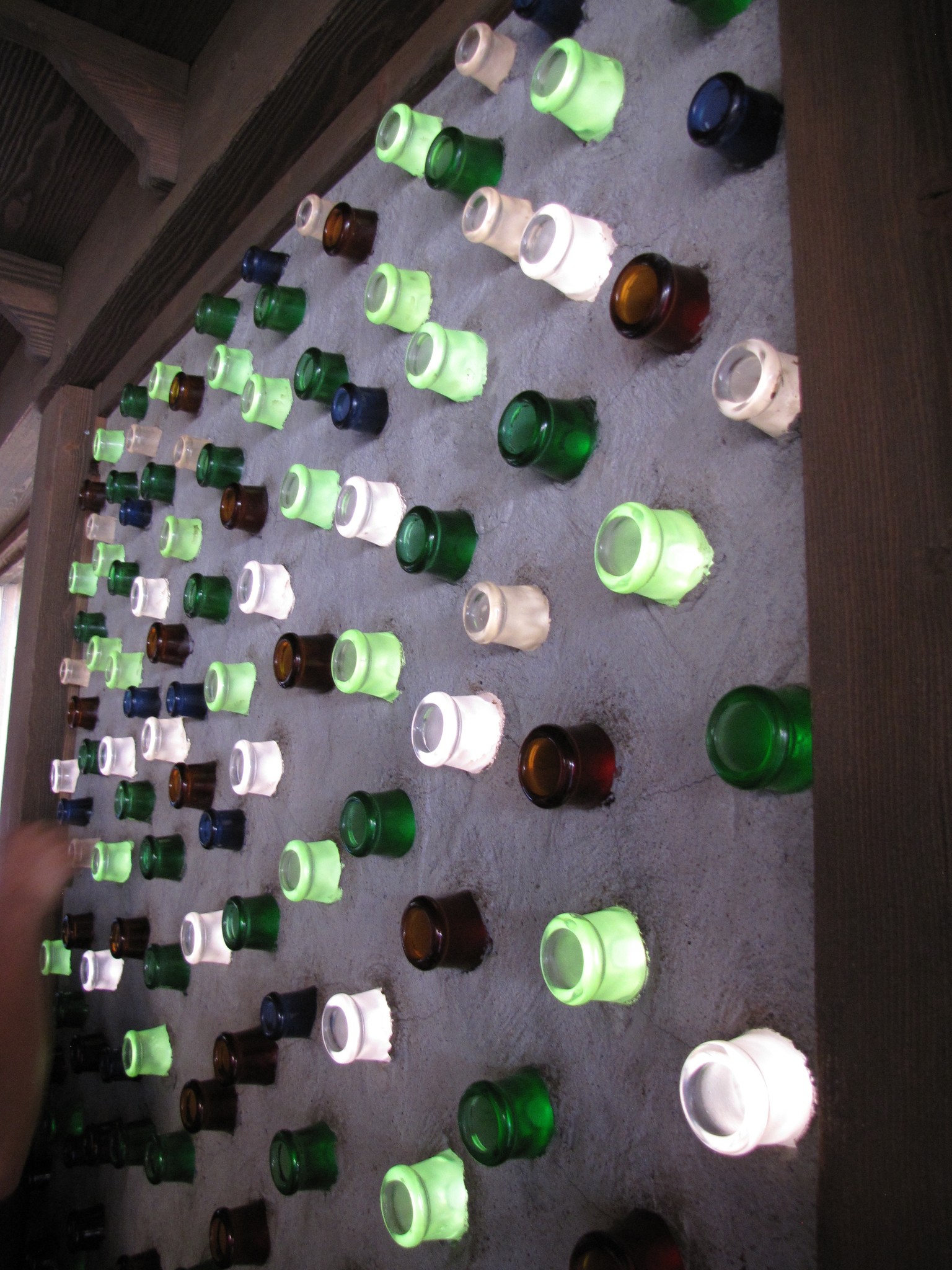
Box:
[594,502,714,606]
[454,1,625,142]
[711,340,801,439]
[569,1209,686,1270]
[704,683,813,794]
[41,345,336,1270]
[279,838,557,1248]
[462,581,551,650]
[516,724,616,810]
[540,906,646,1006]
[498,390,598,485]
[671,0,757,28]
[687,72,784,171]
[678,1028,816,1156]
[331,323,505,855]
[193,196,431,342]
[374,103,711,354]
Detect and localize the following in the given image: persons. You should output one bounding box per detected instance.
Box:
[0,821,87,1207]
